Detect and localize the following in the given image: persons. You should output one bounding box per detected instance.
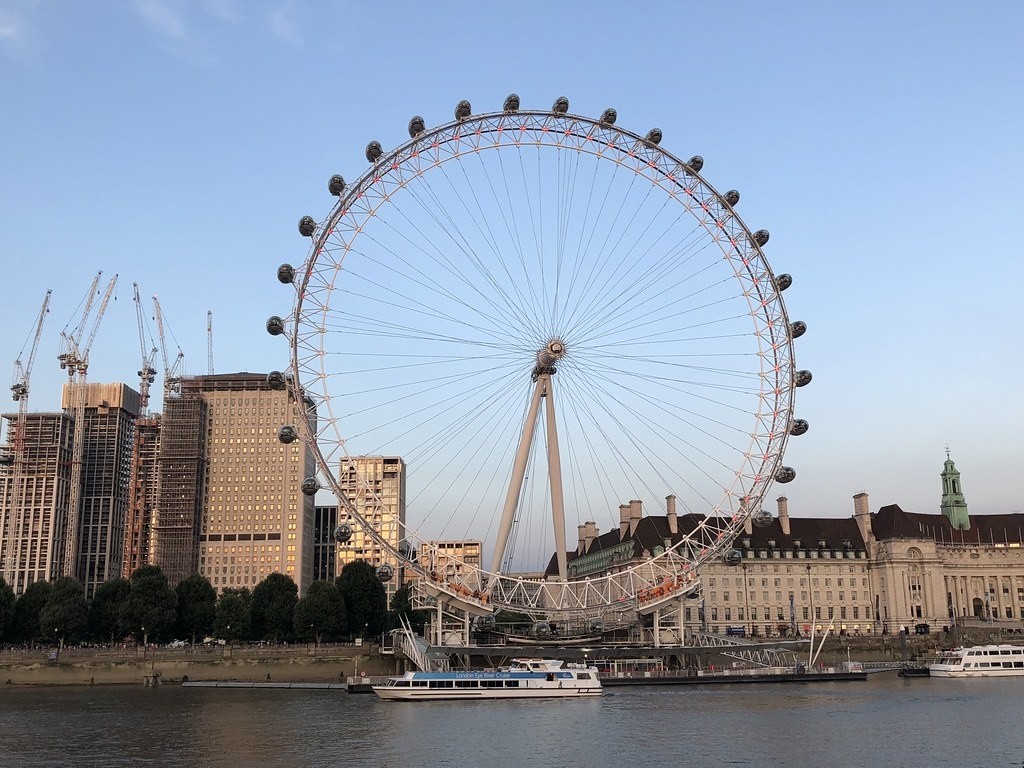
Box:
[754,631,865,638]
[3,639,276,653]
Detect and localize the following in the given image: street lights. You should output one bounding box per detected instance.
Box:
[52,627,63,663]
[226,624,235,660]
[139,626,153,659]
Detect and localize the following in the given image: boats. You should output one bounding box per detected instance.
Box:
[897,665,930,677]
[371,658,604,700]
[929,644,1024,677]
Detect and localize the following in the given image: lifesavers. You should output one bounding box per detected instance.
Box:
[360,671,366,677]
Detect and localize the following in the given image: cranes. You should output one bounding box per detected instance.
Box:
[206,309,216,375]
[57,269,119,374]
[10,287,52,395]
[130,280,159,381]
[151,293,186,383]
